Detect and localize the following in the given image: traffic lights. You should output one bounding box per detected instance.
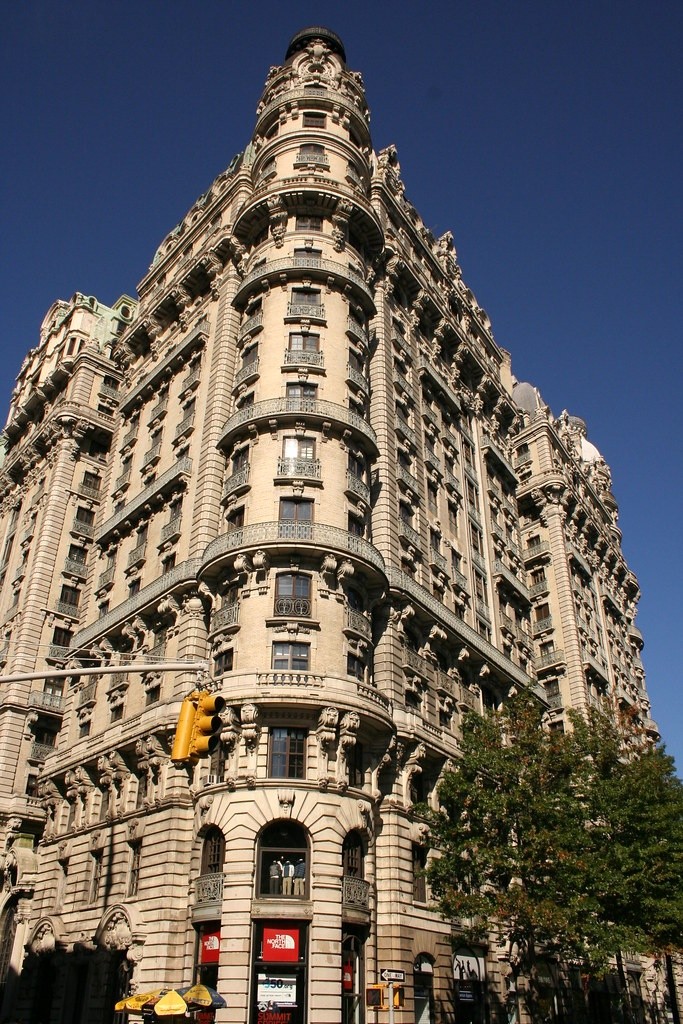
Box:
[393,987,404,1007]
[365,987,384,1007]
[189,689,225,759]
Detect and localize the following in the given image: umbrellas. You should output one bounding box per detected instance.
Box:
[174,982,227,1009]
[114,987,189,1016]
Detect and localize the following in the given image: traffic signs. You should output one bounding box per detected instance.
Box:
[380,969,405,982]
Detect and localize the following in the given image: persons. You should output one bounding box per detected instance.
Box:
[269,859,306,896]
